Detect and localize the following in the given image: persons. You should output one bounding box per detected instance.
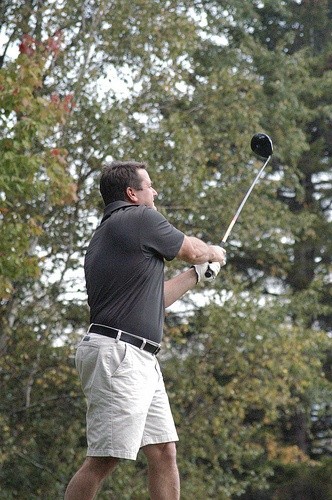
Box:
[62,162,228,500]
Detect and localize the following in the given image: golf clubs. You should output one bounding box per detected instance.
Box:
[204,132,275,277]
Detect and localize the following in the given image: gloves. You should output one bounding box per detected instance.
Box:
[193,259,227,286]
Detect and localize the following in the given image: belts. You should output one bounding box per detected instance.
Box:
[89,324,161,356]
